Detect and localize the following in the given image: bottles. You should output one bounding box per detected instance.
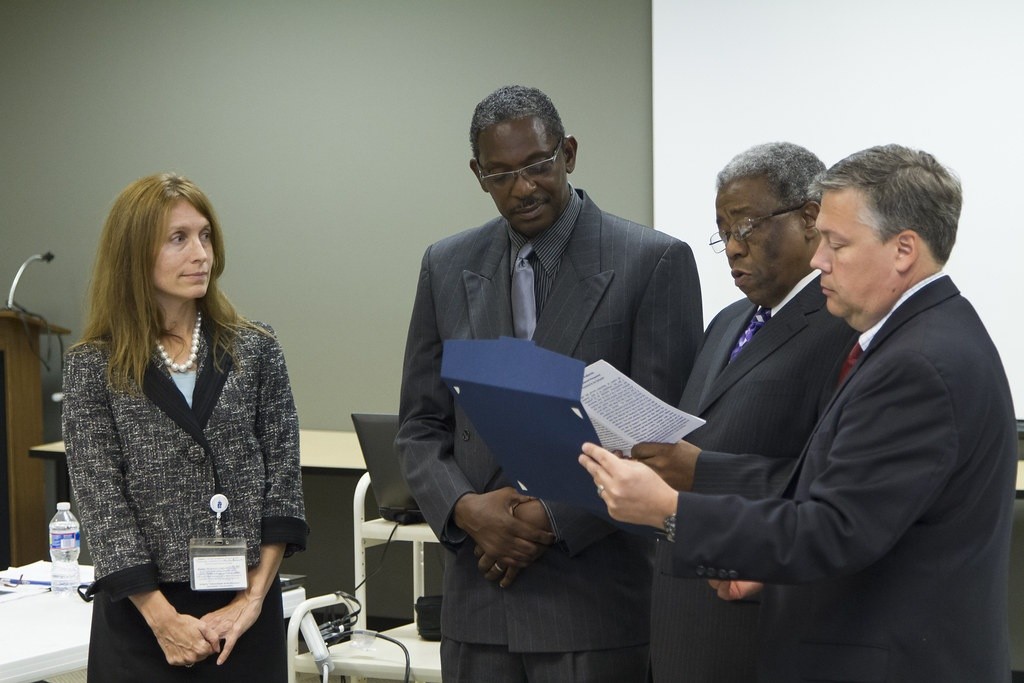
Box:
[49,503,81,591]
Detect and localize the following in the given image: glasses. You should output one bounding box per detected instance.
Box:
[709,199,809,254]
[476,138,566,191]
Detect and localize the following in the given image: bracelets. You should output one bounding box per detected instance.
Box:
[664,512,676,544]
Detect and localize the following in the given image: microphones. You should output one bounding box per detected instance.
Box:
[7,253,55,312]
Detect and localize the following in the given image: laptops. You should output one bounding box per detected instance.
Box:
[352,413,427,525]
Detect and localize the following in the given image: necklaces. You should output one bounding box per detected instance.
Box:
[156,309,201,373]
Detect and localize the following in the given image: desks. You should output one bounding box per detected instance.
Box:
[0,557,100,683]
[23,424,369,472]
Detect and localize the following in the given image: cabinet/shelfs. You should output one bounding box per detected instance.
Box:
[287,471,441,683]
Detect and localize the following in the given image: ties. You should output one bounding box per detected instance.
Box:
[840,341,862,387]
[729,303,771,364]
[510,243,536,341]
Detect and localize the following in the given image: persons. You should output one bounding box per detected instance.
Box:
[62,171,312,683]
[396,84,705,683]
[612,143,858,683]
[579,144,1019,683]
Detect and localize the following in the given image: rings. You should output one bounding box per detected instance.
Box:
[495,562,504,573]
[597,484,604,497]
